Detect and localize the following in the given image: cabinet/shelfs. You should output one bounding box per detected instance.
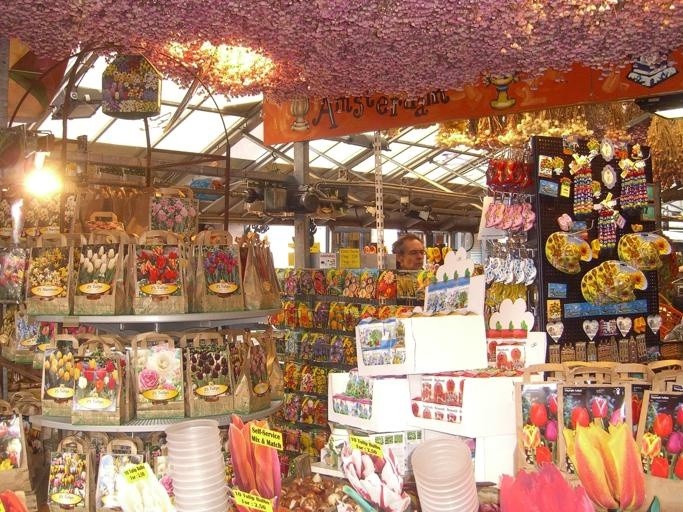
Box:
[275,267,436,473]
[0,224,284,512]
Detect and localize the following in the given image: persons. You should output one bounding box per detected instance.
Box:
[392,234,425,269]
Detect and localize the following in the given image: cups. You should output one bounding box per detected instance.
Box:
[162,417,230,511]
[410,436,479,512]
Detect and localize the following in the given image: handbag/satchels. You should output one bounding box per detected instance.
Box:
[70,336,134,426]
[146,432,176,510]
[131,230,191,315]
[252,332,286,403]
[235,231,283,310]
[96,437,149,511]
[225,329,272,414]
[0,390,46,491]
[2,306,58,370]
[72,227,138,315]
[516,359,683,479]
[42,334,90,417]
[179,332,235,416]
[186,231,246,312]
[26,230,79,317]
[58,185,199,233]
[46,433,97,512]
[219,429,237,488]
[128,332,185,421]
[1,230,32,304]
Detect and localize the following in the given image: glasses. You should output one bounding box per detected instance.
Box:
[409,250,426,255]
